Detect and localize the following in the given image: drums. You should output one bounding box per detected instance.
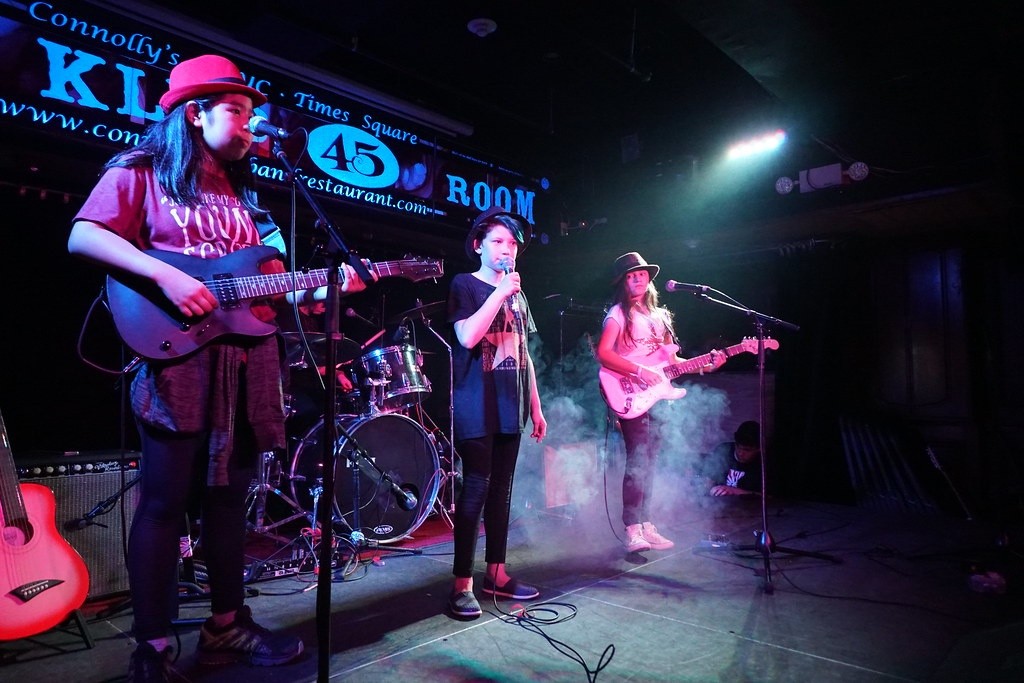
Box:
[340,344,432,415]
[299,336,364,402]
[290,414,441,544]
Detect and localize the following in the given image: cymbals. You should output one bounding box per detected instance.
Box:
[421,350,437,355]
[281,329,357,356]
[384,300,448,324]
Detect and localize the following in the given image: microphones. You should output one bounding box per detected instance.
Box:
[498,257,521,322]
[62,518,94,531]
[399,488,417,510]
[345,308,378,328]
[666,280,712,292]
[394,320,406,341]
[249,115,290,141]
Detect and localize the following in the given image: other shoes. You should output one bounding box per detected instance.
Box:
[481,577,539,600]
[449,588,482,616]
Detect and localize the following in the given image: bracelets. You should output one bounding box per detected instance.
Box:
[637,365,642,379]
[699,366,704,376]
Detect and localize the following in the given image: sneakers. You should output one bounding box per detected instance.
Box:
[625,523,650,553]
[196,606,304,666]
[641,521,675,551]
[127,642,178,683]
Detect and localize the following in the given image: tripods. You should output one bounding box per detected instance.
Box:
[692,290,845,597]
[235,451,325,549]
[335,423,423,581]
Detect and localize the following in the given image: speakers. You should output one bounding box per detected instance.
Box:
[536,437,598,508]
[11,450,144,604]
[544,308,613,440]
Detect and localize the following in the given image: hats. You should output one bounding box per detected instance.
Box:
[609,251,660,287]
[465,206,532,263]
[159,54,267,114]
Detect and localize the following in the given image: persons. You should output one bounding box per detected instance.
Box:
[596,252,726,554]
[67,55,379,683]
[695,420,777,500]
[447,206,547,616]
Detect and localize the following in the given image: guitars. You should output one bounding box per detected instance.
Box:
[598,334,780,420]
[105,247,446,365]
[0,415,91,641]
